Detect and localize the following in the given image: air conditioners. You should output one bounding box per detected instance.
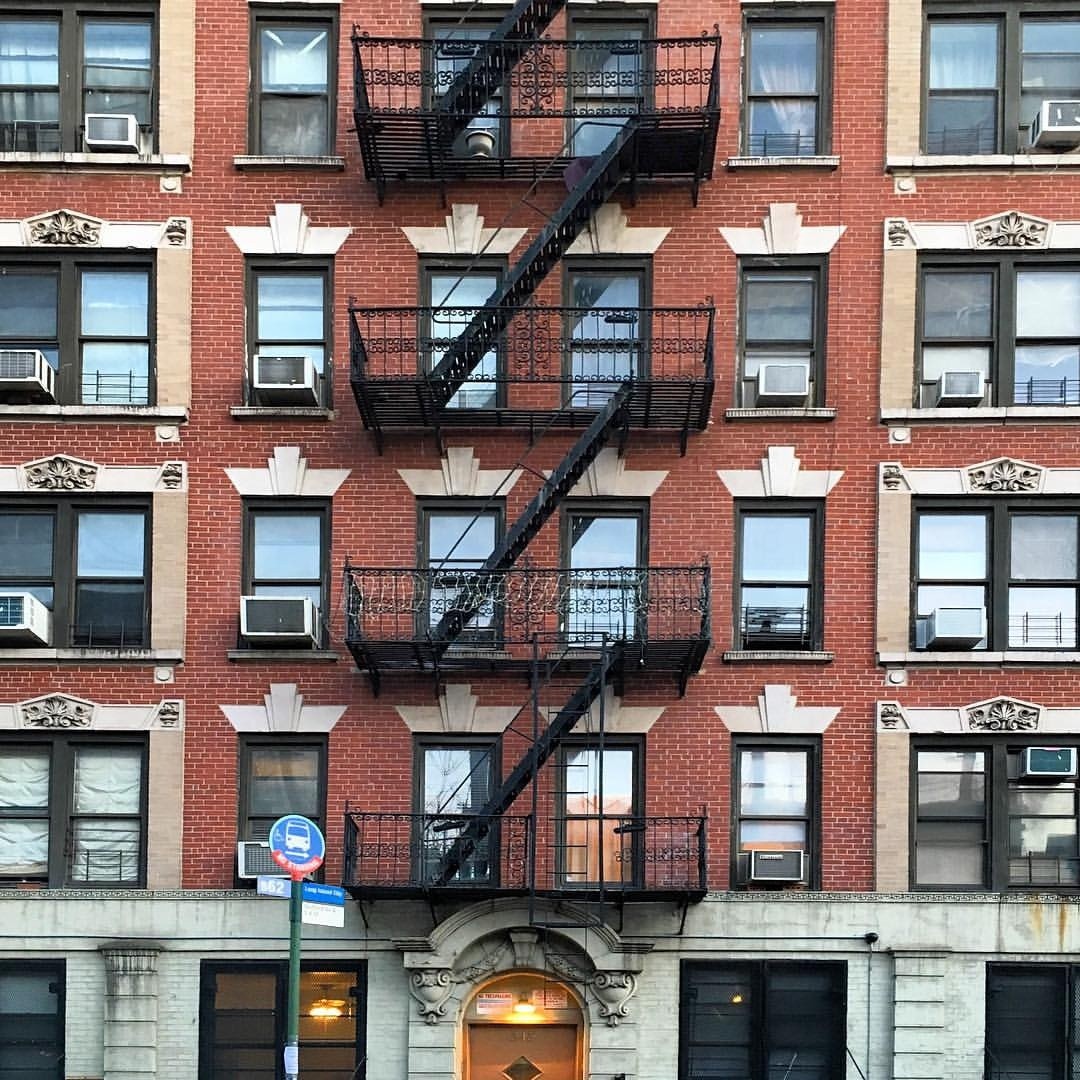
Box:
[85,113,140,154]
[1031,100,1080,148]
[0,592,49,647]
[748,850,803,884]
[237,841,317,882]
[0,349,55,402]
[253,355,320,407]
[936,371,984,408]
[755,364,809,409]
[1021,746,1076,778]
[240,595,321,650]
[927,606,986,650]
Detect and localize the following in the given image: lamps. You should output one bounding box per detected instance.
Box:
[307,985,346,1033]
[513,991,535,1013]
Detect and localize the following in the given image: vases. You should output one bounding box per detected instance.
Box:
[465,130,497,158]
